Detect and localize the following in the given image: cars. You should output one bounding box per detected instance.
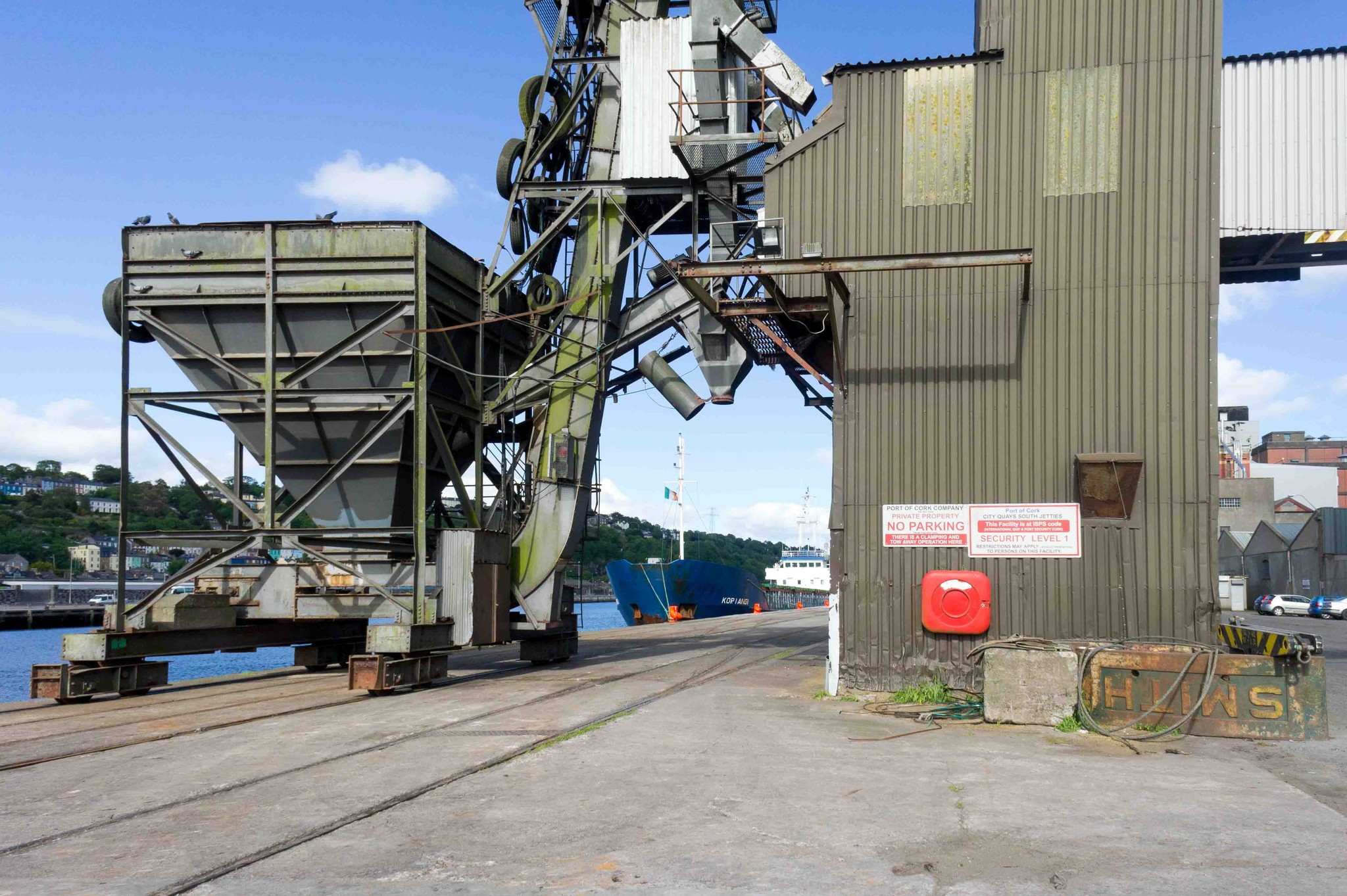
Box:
[1307,596,1344,619]
[1254,595,1270,615]
[1261,594,1311,616]
[1322,596,1347,620]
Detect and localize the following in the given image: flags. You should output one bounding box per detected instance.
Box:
[665,486,678,501]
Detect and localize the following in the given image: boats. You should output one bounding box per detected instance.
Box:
[765,487,831,591]
[605,433,769,628]
